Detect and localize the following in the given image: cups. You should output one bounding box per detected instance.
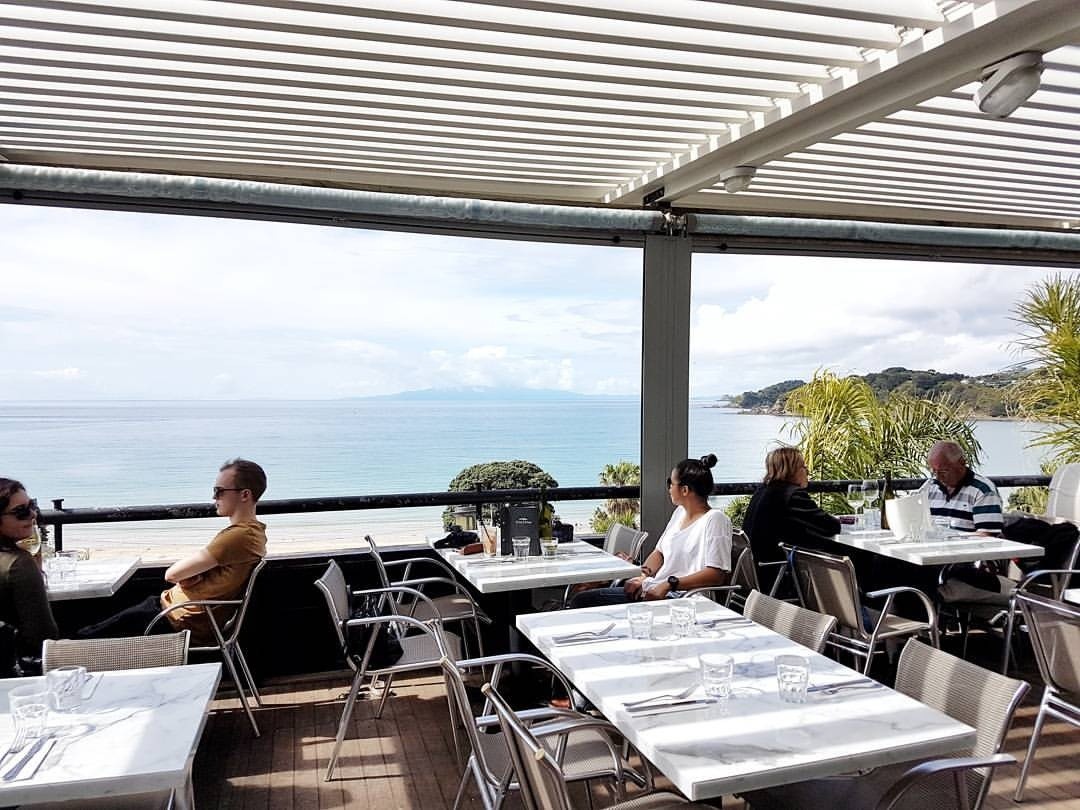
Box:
[55,549,78,582]
[669,598,696,638]
[698,652,734,698]
[512,536,531,561]
[540,536,559,560]
[627,603,654,640]
[934,517,950,530]
[774,653,811,705]
[480,525,497,557]
[47,665,85,712]
[477,518,492,534]
[863,507,881,532]
[7,684,48,739]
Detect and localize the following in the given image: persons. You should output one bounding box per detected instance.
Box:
[744,448,890,612]
[546,455,732,717]
[891,438,1010,641]
[0,479,58,679]
[81,460,267,650]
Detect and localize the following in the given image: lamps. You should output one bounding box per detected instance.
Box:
[973,51,1046,121]
[720,166,757,194]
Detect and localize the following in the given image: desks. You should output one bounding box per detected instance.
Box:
[817,515,1045,565]
[428,538,642,676]
[516,596,977,810]
[0,663,223,810]
[43,557,140,601]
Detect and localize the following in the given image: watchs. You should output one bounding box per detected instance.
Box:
[668,576,679,592]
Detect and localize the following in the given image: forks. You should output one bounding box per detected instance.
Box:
[622,683,697,706]
[811,683,883,695]
[0,728,26,766]
[551,622,616,639]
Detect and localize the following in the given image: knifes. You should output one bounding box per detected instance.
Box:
[3,735,48,780]
[626,699,717,712]
[807,678,872,692]
[555,634,627,644]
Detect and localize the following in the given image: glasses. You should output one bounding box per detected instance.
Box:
[213,487,240,496]
[5,498,38,520]
[666,478,680,490]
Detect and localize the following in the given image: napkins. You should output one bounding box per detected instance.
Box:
[549,634,618,646]
[0,739,57,783]
[624,697,709,717]
[82,672,104,699]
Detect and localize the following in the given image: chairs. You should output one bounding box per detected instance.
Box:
[43,515,1080,810]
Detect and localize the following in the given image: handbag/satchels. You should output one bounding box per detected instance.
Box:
[348,609,405,670]
[885,489,930,544]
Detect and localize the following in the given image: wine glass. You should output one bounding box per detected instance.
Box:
[862,479,879,508]
[846,484,865,531]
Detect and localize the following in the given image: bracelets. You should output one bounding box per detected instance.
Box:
[641,566,652,576]
